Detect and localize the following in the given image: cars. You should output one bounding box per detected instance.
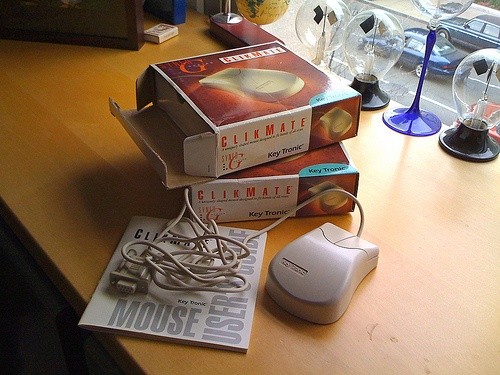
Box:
[359,27,475,81]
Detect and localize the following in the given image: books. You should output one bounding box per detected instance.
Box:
[79,215,268,355]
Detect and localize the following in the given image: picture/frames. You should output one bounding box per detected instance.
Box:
[0,0,145,50]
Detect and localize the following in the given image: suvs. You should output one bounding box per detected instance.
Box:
[426,12,500,53]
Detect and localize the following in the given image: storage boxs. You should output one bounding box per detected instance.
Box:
[188,142,363,222]
[145,24,179,44]
[108,41,362,191]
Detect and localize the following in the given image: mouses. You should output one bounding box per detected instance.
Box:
[266,222,380,324]
[319,106,354,140]
[308,180,349,212]
[199,68,305,101]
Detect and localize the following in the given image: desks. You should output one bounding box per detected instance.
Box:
[0,10,500,375]
